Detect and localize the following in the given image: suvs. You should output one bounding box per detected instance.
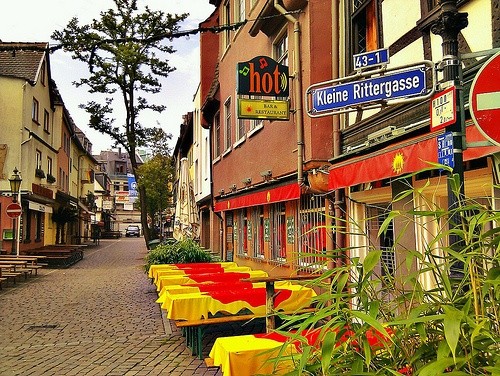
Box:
[125,225,141,237]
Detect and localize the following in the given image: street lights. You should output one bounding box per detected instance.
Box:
[8,166,23,264]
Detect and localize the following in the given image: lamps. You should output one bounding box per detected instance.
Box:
[242,177,252,183]
[218,189,225,194]
[229,183,236,189]
[261,170,271,176]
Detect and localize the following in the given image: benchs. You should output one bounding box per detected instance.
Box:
[174,307,323,359]
[0,250,85,289]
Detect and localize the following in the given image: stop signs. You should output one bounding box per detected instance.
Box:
[6,203,21,218]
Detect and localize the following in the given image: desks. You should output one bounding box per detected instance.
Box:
[25,250,71,267]
[0,255,46,277]
[0,257,37,281]
[36,247,76,265]
[47,244,88,261]
[0,261,27,284]
[148,261,407,376]
[0,265,16,284]
